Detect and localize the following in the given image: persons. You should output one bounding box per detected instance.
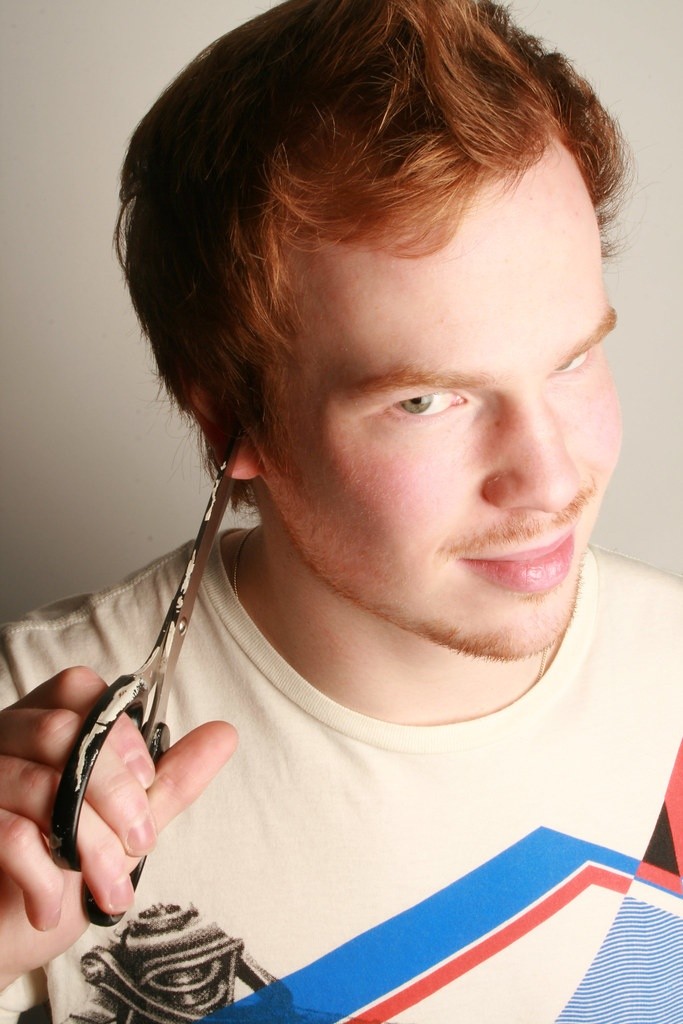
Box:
[0,0,682,1024]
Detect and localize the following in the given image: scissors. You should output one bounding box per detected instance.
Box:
[48,426,247,929]
